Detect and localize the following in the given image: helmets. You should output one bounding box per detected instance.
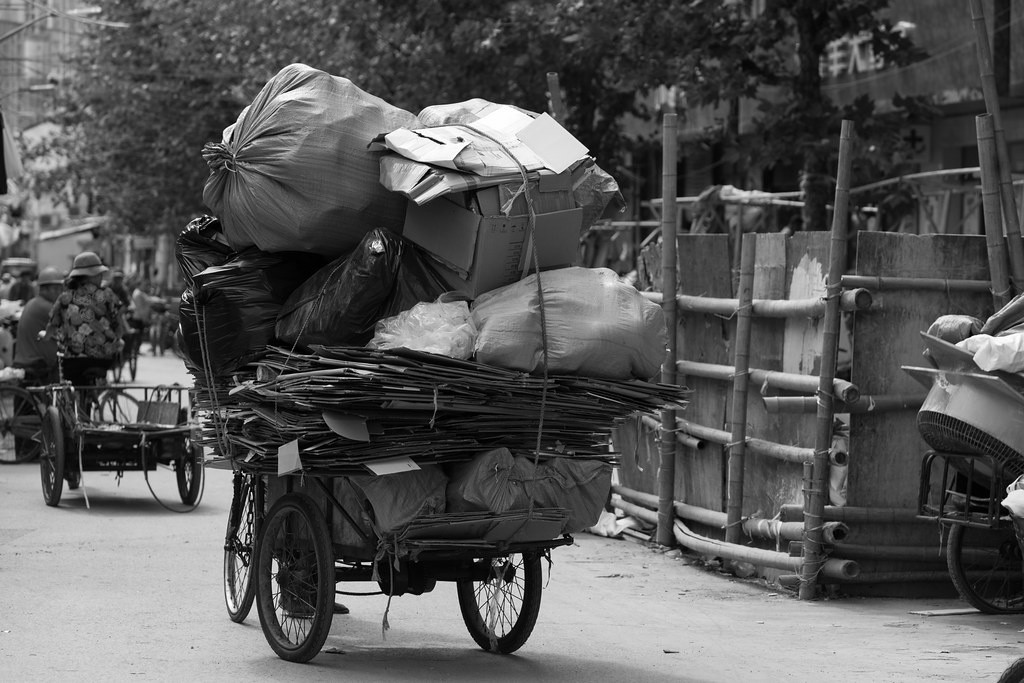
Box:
[37,266,65,285]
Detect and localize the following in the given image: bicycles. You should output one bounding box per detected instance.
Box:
[123,307,167,382]
[0,337,141,470]
[917,450,1024,615]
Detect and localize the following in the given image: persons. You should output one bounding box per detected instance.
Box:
[0,251,168,463]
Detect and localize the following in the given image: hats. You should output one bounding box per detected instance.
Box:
[112,267,123,278]
[68,252,109,277]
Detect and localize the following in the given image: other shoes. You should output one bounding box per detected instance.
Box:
[278,586,350,619]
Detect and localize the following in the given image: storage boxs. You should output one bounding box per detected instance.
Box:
[400,196,584,301]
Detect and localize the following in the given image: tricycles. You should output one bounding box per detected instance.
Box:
[25,333,203,507]
[222,466,575,664]
